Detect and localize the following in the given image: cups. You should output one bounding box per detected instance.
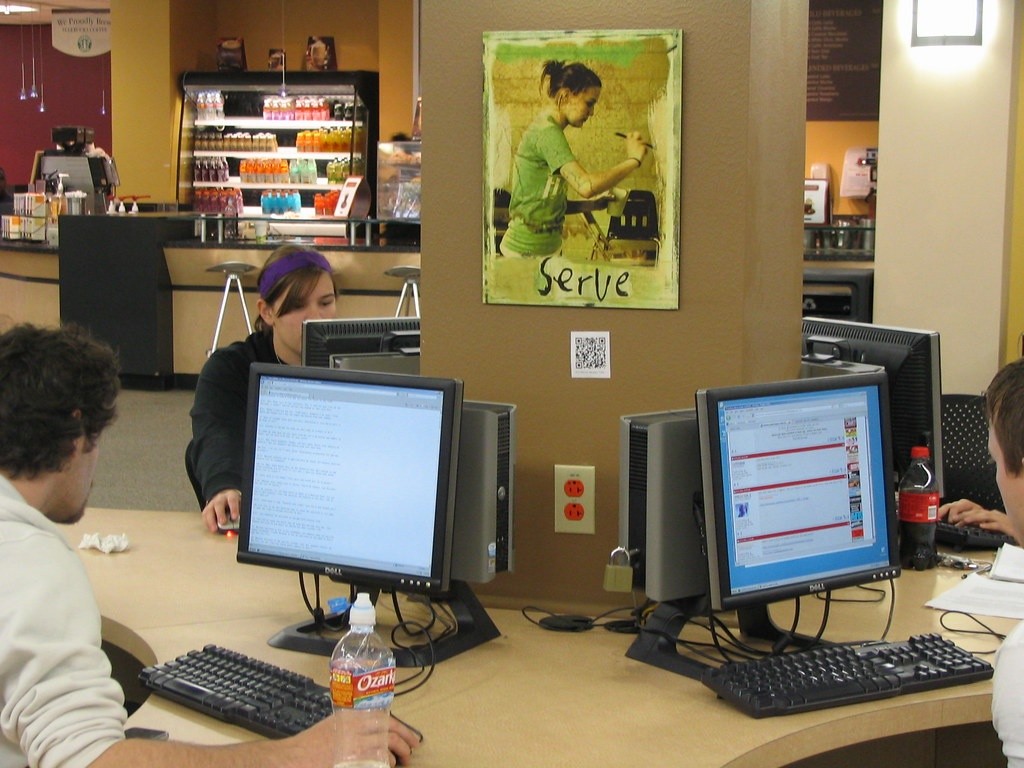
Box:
[607,184,631,217]
[254,219,268,243]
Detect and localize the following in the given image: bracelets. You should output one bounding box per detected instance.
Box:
[630,157,641,167]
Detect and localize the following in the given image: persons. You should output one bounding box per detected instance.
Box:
[499,60,647,261]
[937,357,1024,768]
[0,324,422,768]
[189,243,341,536]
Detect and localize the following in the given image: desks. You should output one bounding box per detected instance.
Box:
[47,507,1024,768]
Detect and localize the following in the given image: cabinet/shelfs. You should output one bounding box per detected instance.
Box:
[174,70,380,249]
[376,139,420,249]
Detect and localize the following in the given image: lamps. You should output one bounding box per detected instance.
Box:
[19,3,44,113]
[101,54,106,116]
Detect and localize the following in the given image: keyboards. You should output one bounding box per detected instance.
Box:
[936,520,1019,549]
[700,633,995,718]
[137,643,332,739]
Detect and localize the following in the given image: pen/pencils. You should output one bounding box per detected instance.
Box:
[961,566,991,579]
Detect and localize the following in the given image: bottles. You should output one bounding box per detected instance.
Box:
[194,131,278,151]
[314,189,341,216]
[326,156,366,185]
[195,188,244,214]
[240,159,318,185]
[261,189,301,214]
[899,446,939,570]
[195,156,230,183]
[328,593,396,768]
[223,197,236,240]
[263,96,366,122]
[195,90,225,121]
[296,126,365,152]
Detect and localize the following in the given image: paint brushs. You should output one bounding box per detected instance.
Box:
[615,132,657,150]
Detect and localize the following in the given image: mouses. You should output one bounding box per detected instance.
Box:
[216,511,242,534]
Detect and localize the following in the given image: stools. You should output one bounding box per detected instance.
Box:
[204,262,257,357]
[384,266,420,318]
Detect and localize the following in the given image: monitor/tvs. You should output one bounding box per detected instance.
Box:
[802,316,950,501]
[236,362,464,595]
[694,372,902,611]
[300,317,421,367]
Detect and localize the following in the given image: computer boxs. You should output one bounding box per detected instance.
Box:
[616,409,708,601]
[329,352,421,375]
[450,400,514,584]
[800,357,887,379]
